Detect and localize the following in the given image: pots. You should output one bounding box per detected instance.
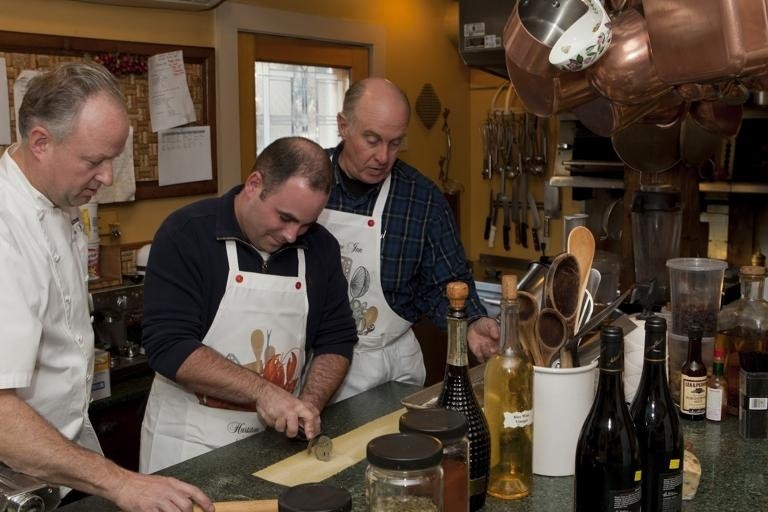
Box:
[501,0,767,188]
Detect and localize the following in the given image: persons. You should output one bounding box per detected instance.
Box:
[0,59,216,512]
[316,77,500,406]
[139,137,360,476]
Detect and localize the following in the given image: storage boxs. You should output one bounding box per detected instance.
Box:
[89,345,113,402]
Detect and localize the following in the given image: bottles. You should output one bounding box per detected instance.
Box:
[738,352,767,438]
[680,320,709,422]
[435,305,492,512]
[630,315,684,512]
[714,264,768,418]
[706,348,728,426]
[362,433,442,511]
[397,407,471,510]
[574,323,644,512]
[92,308,145,359]
[482,297,534,501]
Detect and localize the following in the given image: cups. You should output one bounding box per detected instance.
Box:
[632,189,685,286]
[88,241,99,278]
[80,203,103,243]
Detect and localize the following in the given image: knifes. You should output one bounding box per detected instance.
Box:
[484,173,562,254]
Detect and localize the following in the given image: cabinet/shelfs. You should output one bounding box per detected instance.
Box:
[87,282,149,399]
